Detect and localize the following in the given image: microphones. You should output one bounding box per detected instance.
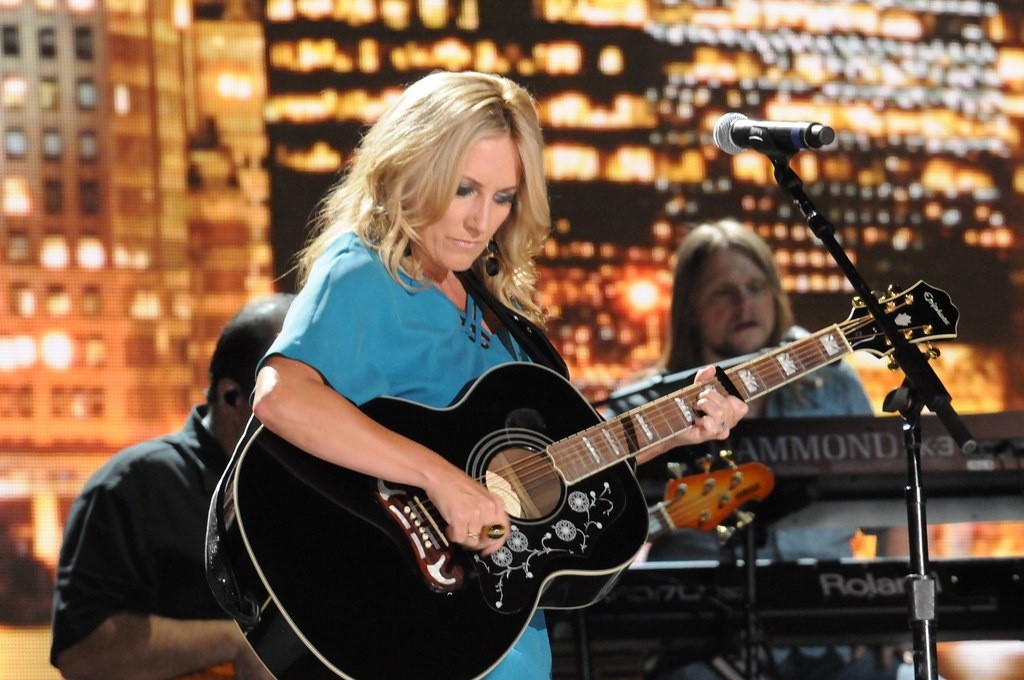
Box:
[712,113,834,154]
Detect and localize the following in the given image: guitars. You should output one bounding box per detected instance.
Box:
[645,450,774,540]
[201,278,963,680]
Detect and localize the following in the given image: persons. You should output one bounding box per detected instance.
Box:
[249,72,748,680]
[604,214,929,679]
[50,292,290,680]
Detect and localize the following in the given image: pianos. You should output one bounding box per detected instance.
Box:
[622,407,1024,508]
[536,554,1024,671]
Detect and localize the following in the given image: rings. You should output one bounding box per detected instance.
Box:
[465,531,480,540]
[718,422,728,434]
[482,523,507,539]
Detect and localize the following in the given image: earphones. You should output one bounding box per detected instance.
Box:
[223,387,239,408]
